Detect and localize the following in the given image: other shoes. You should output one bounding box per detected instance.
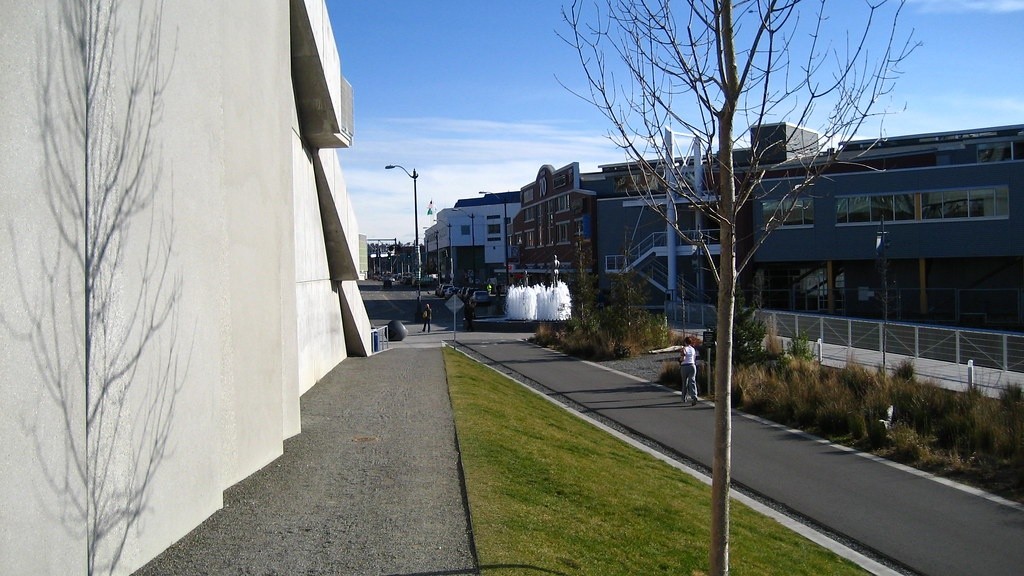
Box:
[692,398,697,406]
[680,399,687,402]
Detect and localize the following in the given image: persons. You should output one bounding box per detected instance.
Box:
[422,303,432,333]
[679,336,698,406]
[551,255,560,288]
[463,303,476,332]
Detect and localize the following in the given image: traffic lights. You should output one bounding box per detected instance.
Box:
[407,253,411,258]
[388,253,391,260]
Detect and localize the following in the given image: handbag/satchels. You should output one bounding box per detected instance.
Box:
[423,310,427,318]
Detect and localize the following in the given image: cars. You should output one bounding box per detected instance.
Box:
[373,271,419,287]
[433,283,492,308]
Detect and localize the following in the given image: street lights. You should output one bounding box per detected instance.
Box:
[451,206,477,288]
[383,164,424,324]
[433,219,452,284]
[422,226,441,285]
[417,236,430,278]
[478,190,510,294]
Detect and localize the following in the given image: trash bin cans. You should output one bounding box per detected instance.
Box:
[371,327,378,352]
